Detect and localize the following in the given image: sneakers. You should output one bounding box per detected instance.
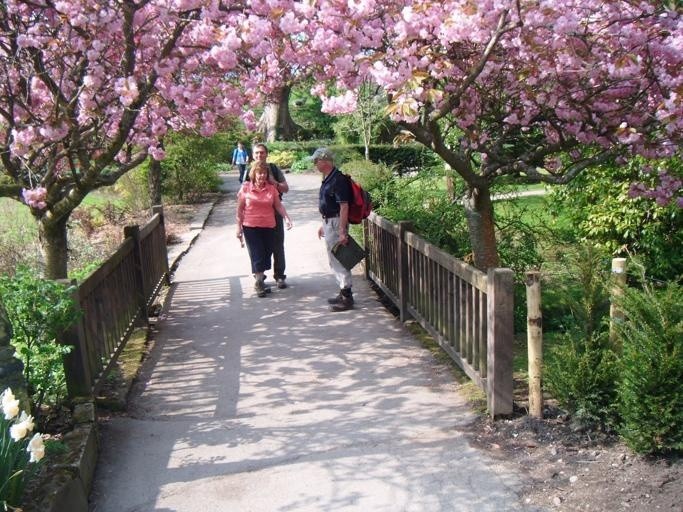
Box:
[253,274,271,297]
[276,278,287,289]
[327,287,354,312]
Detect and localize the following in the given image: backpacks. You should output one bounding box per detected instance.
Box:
[330,172,372,225]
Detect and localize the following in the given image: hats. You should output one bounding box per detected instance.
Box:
[305,148,334,161]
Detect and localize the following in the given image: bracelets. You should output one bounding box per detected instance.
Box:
[276,183,280,190]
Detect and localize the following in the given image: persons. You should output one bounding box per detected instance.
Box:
[308,145,355,310]
[240,143,292,293]
[232,160,288,299]
[229,139,249,181]
